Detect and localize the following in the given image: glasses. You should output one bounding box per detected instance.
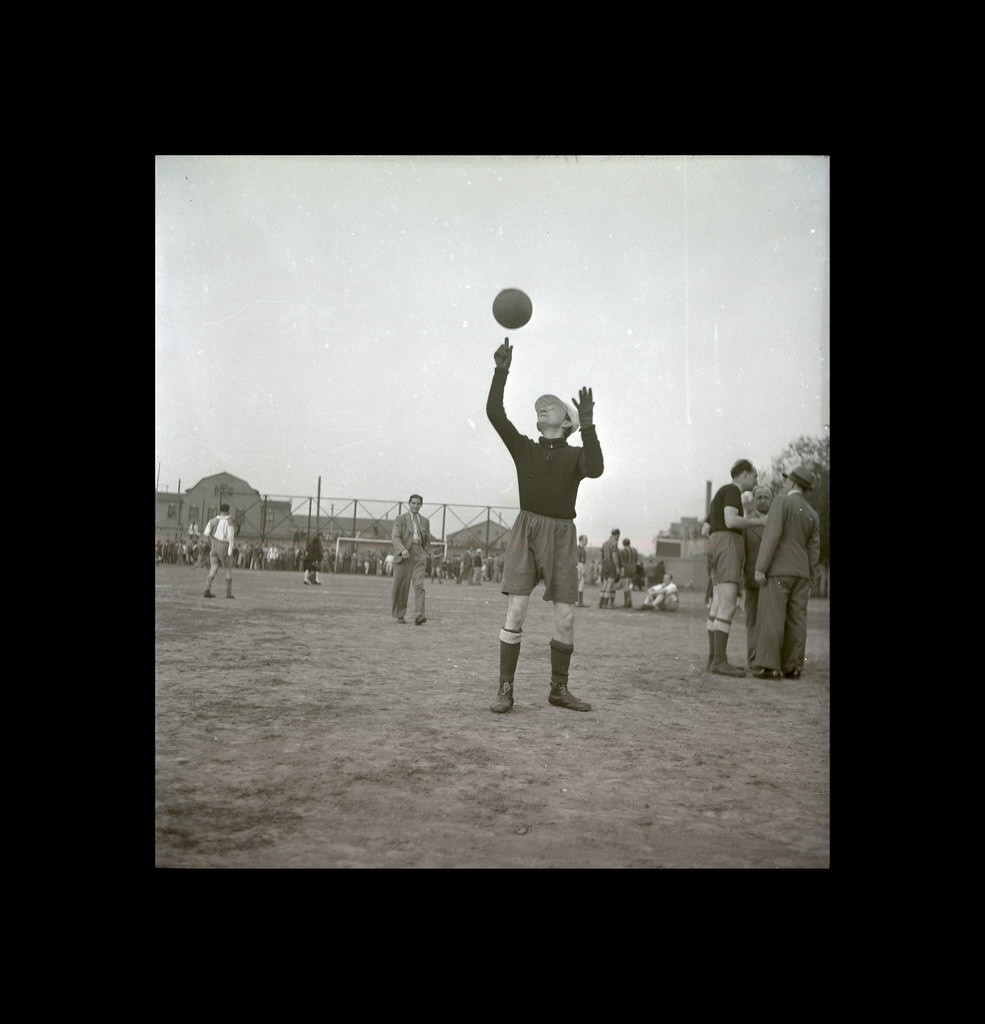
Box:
[547,405,564,420]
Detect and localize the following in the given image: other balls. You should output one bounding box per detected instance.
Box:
[492,288,533,330]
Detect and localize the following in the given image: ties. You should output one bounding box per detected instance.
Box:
[414,516,422,546]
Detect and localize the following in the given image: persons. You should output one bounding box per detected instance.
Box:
[156,521,395,585]
[701,459,768,677]
[203,504,235,598]
[426,546,505,585]
[741,485,774,668]
[754,467,820,681]
[592,530,665,609]
[640,574,678,611]
[576,535,589,607]
[389,494,429,625]
[486,338,604,713]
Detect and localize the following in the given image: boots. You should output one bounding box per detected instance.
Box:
[315,571,321,585]
[204,590,216,598]
[304,570,312,585]
[226,589,235,598]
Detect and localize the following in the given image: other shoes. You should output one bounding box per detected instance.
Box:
[548,681,591,711]
[577,601,662,612]
[397,617,405,623]
[754,668,782,679]
[783,669,800,678]
[707,660,746,677]
[415,615,426,625]
[490,681,514,712]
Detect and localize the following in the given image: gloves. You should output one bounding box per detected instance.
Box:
[572,386,595,426]
[494,337,513,370]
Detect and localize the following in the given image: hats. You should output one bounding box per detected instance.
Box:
[782,467,814,490]
[535,395,580,435]
